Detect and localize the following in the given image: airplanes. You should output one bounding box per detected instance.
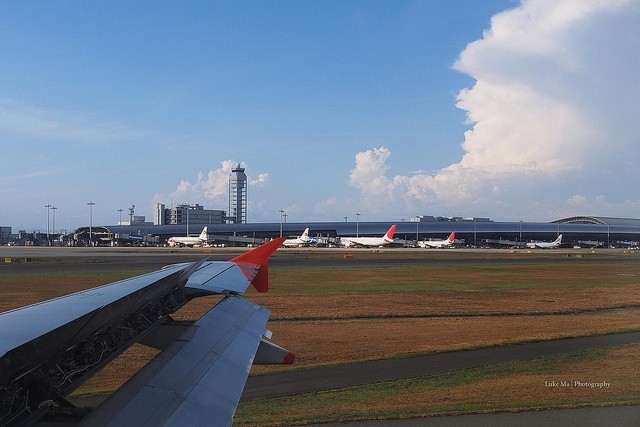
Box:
[270,227,311,247]
[341,224,399,247]
[167,225,209,247]
[525,233,563,249]
[416,231,456,248]
[0,234,294,427]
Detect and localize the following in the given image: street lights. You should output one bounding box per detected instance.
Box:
[88,202,95,239]
[185,206,189,237]
[283,214,288,223]
[118,209,123,227]
[45,204,52,239]
[344,216,348,222]
[51,207,57,237]
[279,210,284,238]
[356,212,360,237]
